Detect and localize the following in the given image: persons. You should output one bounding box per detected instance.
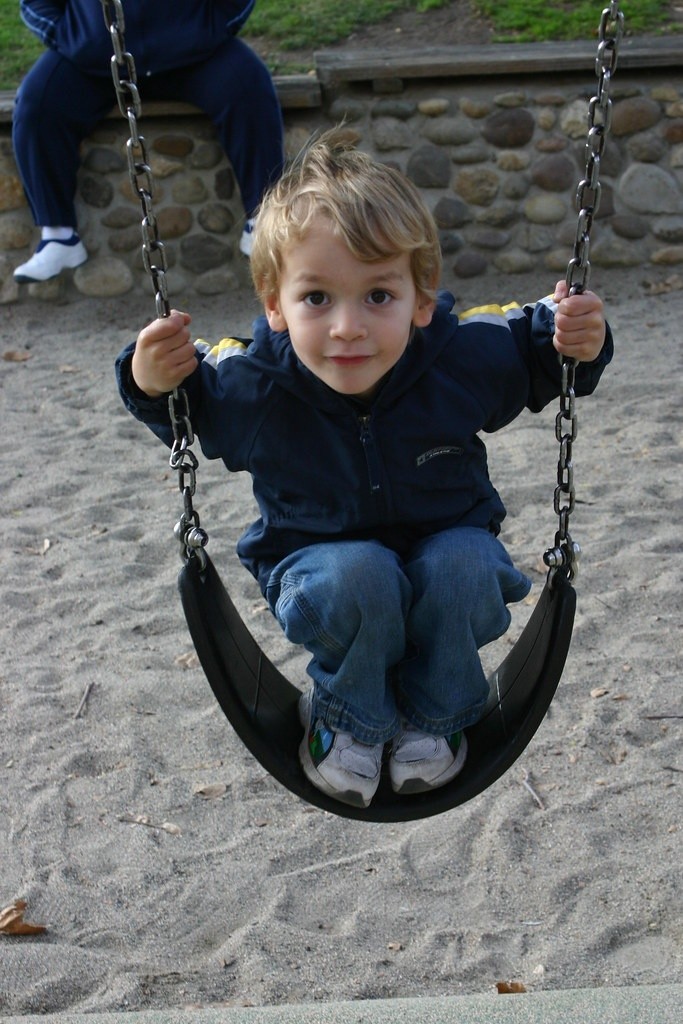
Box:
[115,147,614,811]
[9,0,286,283]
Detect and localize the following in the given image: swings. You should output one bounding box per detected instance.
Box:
[102,0,624,823]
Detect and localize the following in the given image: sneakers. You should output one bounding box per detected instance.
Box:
[387,708,468,795]
[12,227,88,283]
[239,219,257,256]
[298,684,385,809]
